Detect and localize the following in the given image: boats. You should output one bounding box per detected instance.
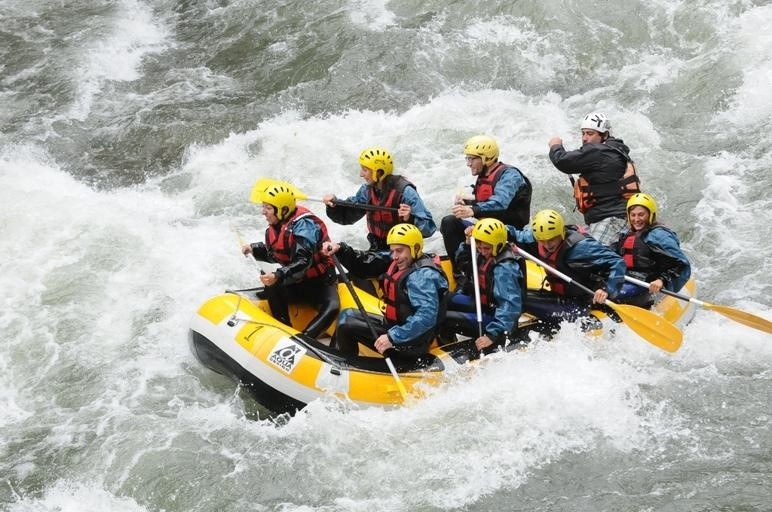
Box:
[188,273,701,417]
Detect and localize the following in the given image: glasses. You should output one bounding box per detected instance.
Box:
[465,156,479,161]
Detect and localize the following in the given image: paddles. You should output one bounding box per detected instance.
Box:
[506,239,683,353]
[248,178,402,213]
[615,268,772,342]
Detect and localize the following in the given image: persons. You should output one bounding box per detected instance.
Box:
[439,217,524,355]
[603,193,691,309]
[320,149,437,251]
[462,209,629,321]
[320,221,451,362]
[439,132,531,265]
[239,176,340,341]
[546,109,640,249]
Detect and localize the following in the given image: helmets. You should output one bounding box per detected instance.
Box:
[626,193,656,225]
[581,112,612,136]
[263,186,296,220]
[359,148,393,182]
[464,136,498,168]
[533,210,565,241]
[387,224,423,259]
[473,218,506,256]
